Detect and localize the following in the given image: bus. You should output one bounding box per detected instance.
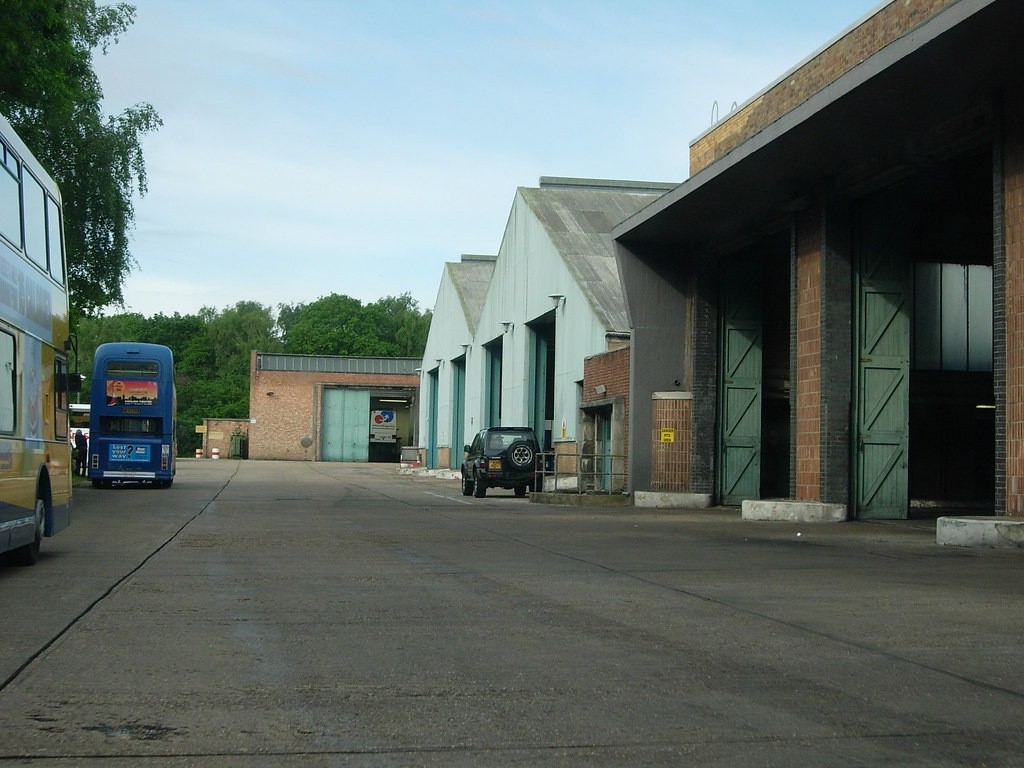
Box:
[67,403,90,476]
[0,116,82,565]
[88,343,178,489]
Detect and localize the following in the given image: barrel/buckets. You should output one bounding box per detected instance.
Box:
[195,448,203,458]
[211,447,219,459]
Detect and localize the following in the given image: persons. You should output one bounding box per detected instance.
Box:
[75,430,87,476]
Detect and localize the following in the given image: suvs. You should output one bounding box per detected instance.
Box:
[460,427,544,501]
